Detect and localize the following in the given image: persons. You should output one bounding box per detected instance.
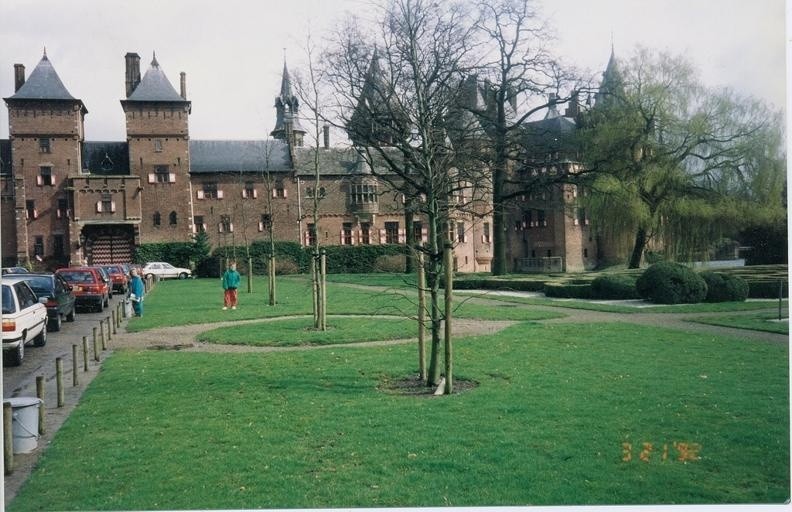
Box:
[130,267,144,317]
[222,262,241,310]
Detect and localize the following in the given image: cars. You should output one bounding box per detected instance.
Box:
[142,261,193,283]
[1,263,145,366]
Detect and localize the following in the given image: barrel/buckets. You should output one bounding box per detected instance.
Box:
[125,301,132,316]
[3,397,41,454]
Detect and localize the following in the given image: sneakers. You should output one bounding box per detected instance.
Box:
[221,305,236,311]
[132,315,143,318]
